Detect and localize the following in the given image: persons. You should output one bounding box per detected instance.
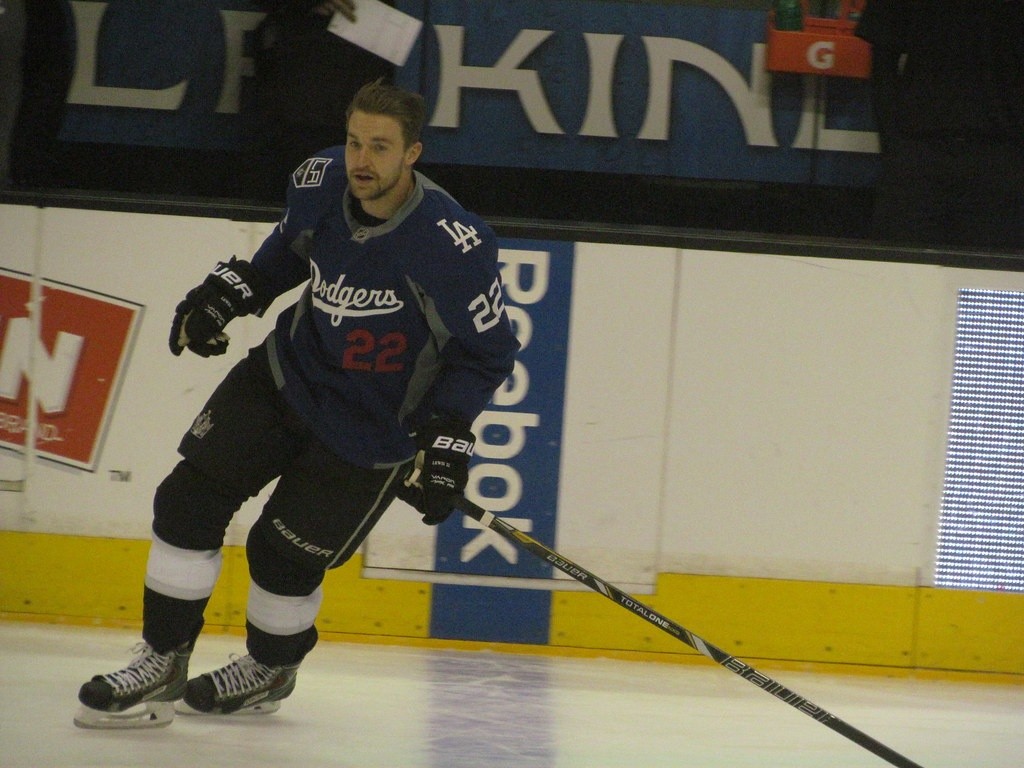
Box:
[72,82,521,730]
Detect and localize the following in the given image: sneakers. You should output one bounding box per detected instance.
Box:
[177,651,304,718]
[74,617,205,731]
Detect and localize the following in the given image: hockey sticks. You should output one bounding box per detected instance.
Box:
[400,465,924,768]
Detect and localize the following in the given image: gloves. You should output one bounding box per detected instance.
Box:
[393,425,475,525]
[169,256,263,358]
